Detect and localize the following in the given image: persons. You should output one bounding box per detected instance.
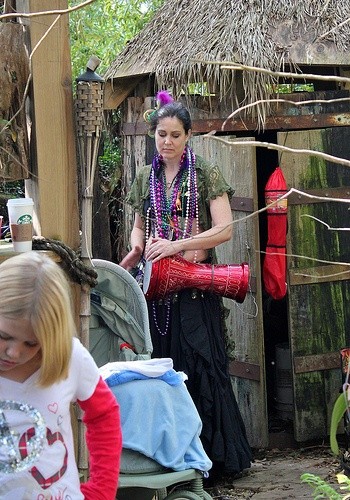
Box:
[120,102,256,488]
[0,251,123,500]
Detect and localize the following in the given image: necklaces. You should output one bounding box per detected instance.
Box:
[135,146,199,336]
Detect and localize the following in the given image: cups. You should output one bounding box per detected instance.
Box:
[6,197,34,253]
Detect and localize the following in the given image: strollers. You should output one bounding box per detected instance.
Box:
[89,257,212,500]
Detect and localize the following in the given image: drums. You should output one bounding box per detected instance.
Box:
[145,254,249,305]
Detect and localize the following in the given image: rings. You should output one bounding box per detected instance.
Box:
[157,250,161,254]
